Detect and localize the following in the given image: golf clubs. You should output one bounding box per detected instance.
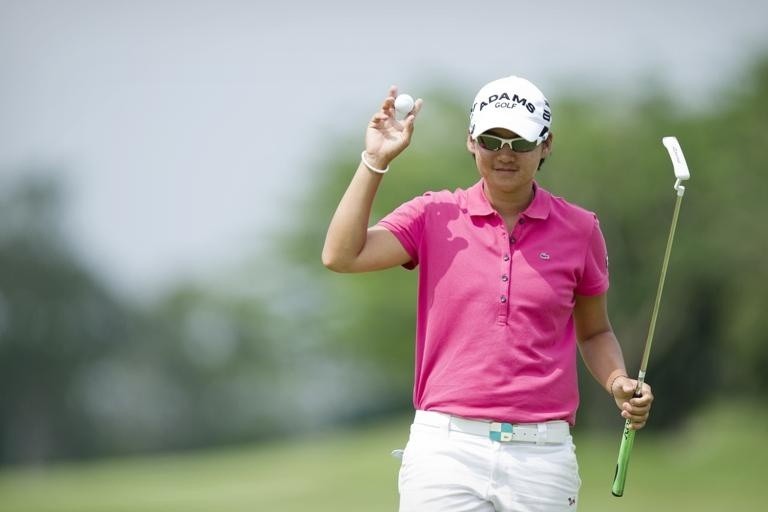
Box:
[613,136,691,498]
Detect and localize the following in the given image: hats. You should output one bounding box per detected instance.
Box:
[469,77,552,142]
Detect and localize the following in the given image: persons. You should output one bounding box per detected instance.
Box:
[320,74,654,512]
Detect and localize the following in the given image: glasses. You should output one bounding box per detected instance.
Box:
[477,132,549,152]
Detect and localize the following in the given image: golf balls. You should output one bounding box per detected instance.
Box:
[395,94,415,113]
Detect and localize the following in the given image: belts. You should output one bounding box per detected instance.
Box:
[413,410,570,443]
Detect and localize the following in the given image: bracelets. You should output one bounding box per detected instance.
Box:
[605,369,629,398]
[360,150,390,174]
